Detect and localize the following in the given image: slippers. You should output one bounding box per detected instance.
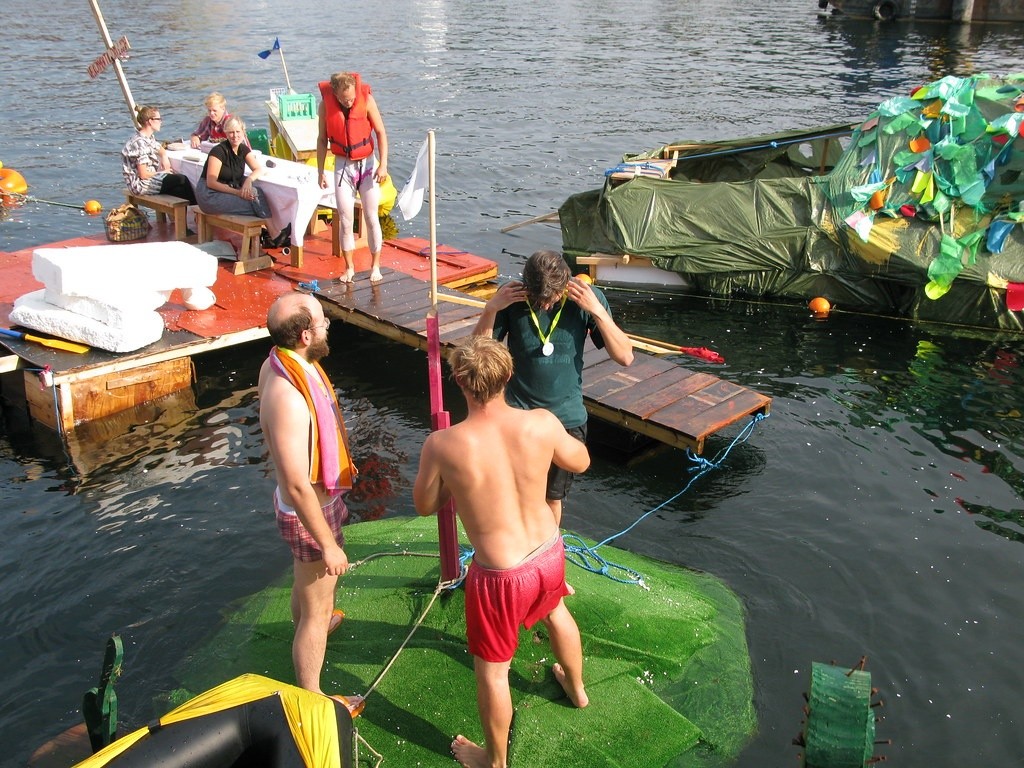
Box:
[331,693,366,719]
[327,610,344,635]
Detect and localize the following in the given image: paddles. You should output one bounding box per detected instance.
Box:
[0,327,91,354]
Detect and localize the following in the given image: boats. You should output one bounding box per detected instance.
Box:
[557,71,1024,333]
[70,672,354,767]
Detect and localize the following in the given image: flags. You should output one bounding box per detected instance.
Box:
[258,37,281,59]
[396,136,429,220]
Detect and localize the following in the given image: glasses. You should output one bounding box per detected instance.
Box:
[334,91,356,104]
[307,317,330,330]
[146,117,162,122]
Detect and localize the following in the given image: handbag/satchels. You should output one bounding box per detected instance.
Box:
[102,203,150,241]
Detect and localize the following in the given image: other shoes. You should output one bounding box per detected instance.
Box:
[273,223,292,247]
[186,228,194,236]
[259,227,275,248]
[270,238,292,248]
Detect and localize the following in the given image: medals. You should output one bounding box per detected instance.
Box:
[542,342,554,356]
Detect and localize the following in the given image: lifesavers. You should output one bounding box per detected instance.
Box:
[0,168,27,199]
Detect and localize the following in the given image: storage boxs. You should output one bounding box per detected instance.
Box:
[278,93,317,121]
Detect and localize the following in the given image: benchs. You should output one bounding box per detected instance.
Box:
[126,193,189,241]
[191,204,275,276]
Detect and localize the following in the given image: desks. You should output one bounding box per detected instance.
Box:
[264,101,335,162]
[156,139,343,268]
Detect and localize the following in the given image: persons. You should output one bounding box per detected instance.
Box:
[259,292,361,714]
[195,115,292,250]
[472,250,635,594]
[413,334,590,768]
[317,71,388,282]
[190,91,251,150]
[121,105,198,238]
